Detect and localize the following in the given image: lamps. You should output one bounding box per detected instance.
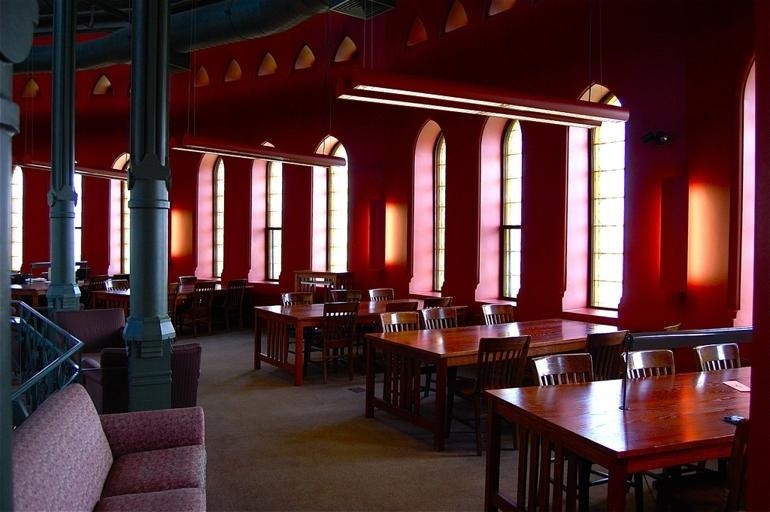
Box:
[336,3,631,129]
[167,0,345,169]
[18,1,129,183]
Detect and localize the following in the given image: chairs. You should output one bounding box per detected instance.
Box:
[529,353,644,510]
[693,343,743,472]
[364,302,691,451]
[12,257,453,387]
[78,343,202,415]
[625,350,711,511]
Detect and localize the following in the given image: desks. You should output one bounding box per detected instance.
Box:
[486,366,752,511]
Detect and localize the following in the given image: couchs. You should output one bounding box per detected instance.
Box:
[9,380,209,512]
